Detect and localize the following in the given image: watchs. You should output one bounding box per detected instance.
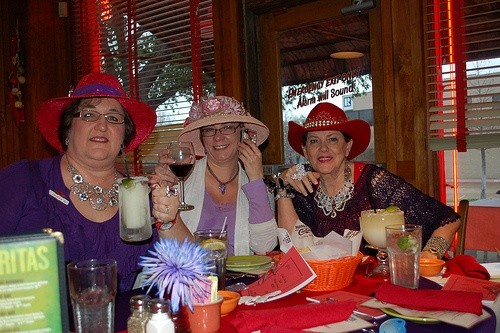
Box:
[153,214,177,231]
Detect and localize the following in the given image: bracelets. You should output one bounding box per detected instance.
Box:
[422,236,449,259]
[272,172,296,200]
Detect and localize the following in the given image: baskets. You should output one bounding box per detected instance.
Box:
[301,251,364,291]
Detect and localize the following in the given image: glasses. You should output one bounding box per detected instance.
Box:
[200,123,241,137]
[72,109,125,124]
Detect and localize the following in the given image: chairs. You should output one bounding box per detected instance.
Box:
[455,199,500,257]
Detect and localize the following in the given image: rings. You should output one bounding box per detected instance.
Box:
[290,164,307,180]
[165,205,169,214]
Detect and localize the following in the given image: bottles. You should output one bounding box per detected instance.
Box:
[145,298,175,333]
[127,294,151,333]
[385,223,422,290]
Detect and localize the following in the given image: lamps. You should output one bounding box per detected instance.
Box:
[330,51,363,59]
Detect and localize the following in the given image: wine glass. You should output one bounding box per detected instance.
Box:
[360,209,405,283]
[167,141,196,211]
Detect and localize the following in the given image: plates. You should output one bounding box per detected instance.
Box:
[380,308,442,324]
[225,255,273,271]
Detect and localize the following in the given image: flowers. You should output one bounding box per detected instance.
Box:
[137,238,218,312]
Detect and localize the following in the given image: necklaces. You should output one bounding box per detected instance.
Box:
[66,154,119,211]
[207,162,239,194]
[314,163,354,218]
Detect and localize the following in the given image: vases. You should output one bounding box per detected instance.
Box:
[186,295,223,333]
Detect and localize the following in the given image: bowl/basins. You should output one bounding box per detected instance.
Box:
[216,291,240,317]
[419,257,446,277]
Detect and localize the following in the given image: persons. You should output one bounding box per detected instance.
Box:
[265,103,461,260]
[154,95,277,258]
[1,73,197,294]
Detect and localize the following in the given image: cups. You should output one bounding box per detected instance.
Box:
[66,257,117,333]
[179,294,223,333]
[117,176,169,243]
[194,230,227,290]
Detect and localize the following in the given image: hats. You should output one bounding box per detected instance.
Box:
[287,102,371,161]
[177,96,270,156]
[35,73,157,156]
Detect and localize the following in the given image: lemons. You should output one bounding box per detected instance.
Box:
[384,206,401,213]
[122,177,137,192]
[397,233,419,249]
[201,239,227,249]
